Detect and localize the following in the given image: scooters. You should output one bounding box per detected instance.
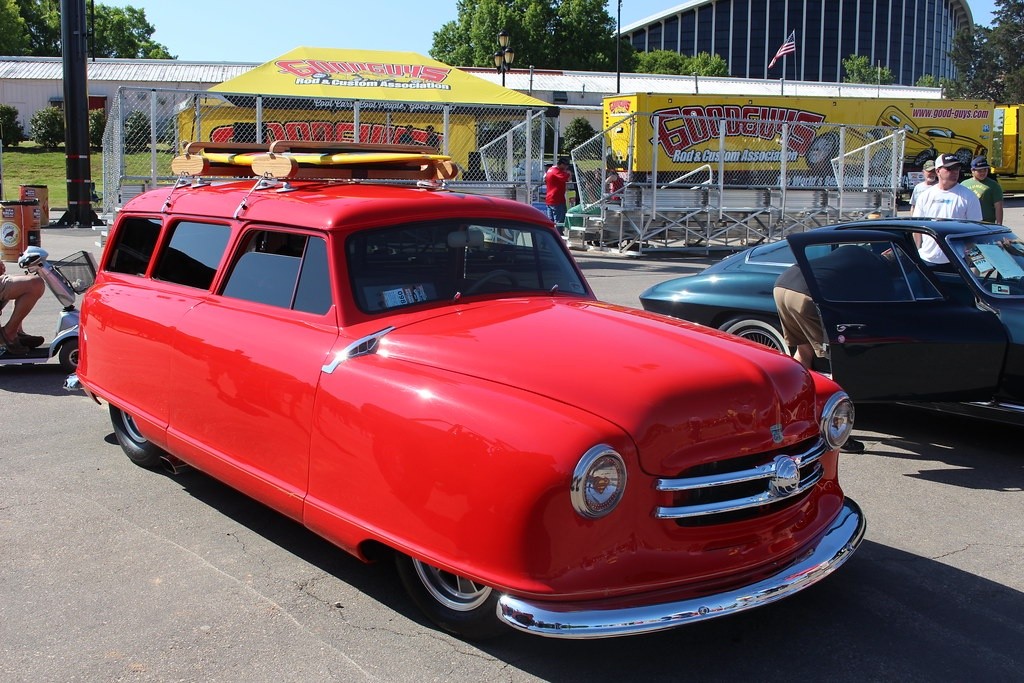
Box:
[0,230,98,373]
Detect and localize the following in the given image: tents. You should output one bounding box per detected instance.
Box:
[174,47,562,181]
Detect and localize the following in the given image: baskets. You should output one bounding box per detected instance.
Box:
[53,251,99,295]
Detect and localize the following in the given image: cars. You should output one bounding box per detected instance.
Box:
[76,140,868,644]
[639,217,1024,407]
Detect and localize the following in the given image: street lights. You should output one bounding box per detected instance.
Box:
[493,29,515,87]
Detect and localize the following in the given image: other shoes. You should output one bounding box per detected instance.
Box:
[0,328,30,355]
[16,330,44,348]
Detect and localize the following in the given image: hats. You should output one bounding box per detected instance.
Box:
[923,160,935,171]
[970,156,990,169]
[934,153,962,170]
[881,248,915,276]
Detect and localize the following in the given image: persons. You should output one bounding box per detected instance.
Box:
[544,157,571,235]
[908,160,939,218]
[1,257,46,359]
[959,155,1003,225]
[510,155,624,205]
[399,124,414,146]
[772,245,910,456]
[425,124,439,150]
[910,152,984,272]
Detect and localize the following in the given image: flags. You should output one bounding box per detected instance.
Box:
[767,32,796,69]
